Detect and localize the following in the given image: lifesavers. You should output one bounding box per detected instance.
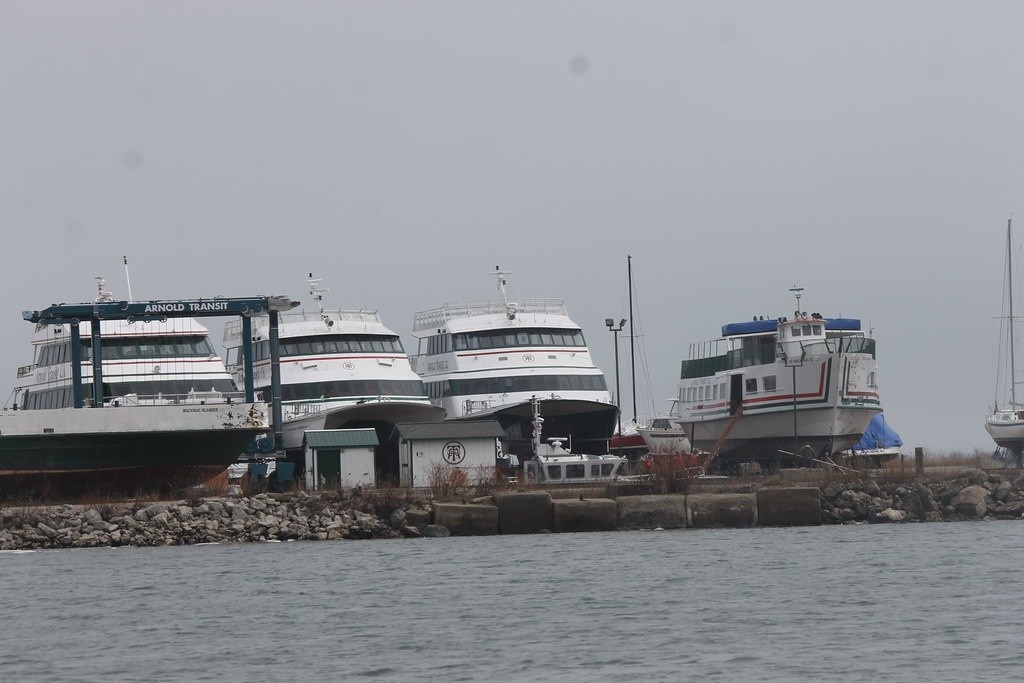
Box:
[244,436,271,454]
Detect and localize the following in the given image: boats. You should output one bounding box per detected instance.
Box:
[675,287,882,467]
[409,264,620,461]
[221,270,444,450]
[0,253,272,501]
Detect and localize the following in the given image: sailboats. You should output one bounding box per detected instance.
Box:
[613,253,693,460]
[984,218,1023,466]
